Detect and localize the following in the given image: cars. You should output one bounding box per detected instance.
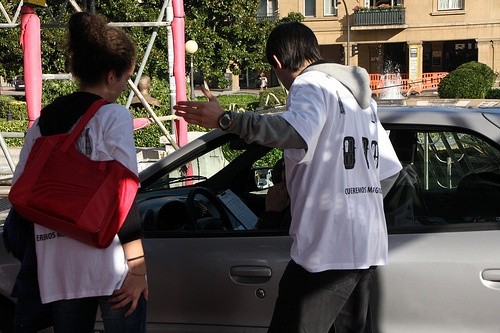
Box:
[0,99,500,333]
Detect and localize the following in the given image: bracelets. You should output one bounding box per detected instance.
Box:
[129,272,147,276]
[127,255,144,261]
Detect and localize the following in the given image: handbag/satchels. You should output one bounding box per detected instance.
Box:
[8,98,142,250]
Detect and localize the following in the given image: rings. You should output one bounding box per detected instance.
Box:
[272,186,277,189]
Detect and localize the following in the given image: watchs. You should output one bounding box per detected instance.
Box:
[218,111,232,131]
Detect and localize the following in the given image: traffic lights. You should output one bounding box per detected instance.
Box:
[352,44,359,56]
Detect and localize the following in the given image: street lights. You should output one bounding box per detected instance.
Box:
[185,40,198,100]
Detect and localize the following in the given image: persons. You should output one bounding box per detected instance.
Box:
[255,180,292,229]
[172,21,402,333]
[10,11,150,333]
[257,72,268,91]
